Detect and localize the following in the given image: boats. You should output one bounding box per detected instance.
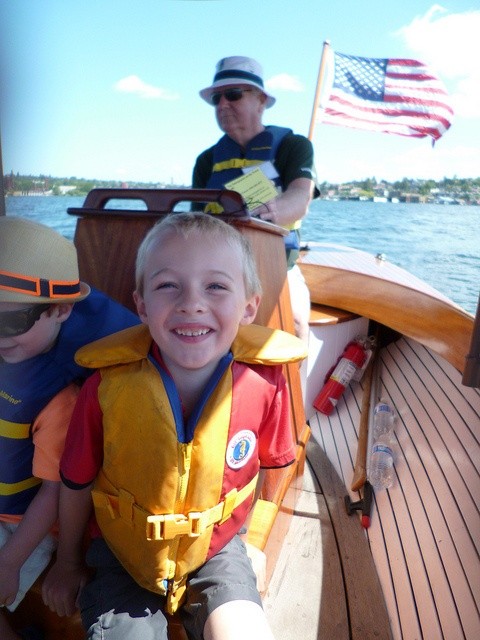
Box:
[65,189,480,639]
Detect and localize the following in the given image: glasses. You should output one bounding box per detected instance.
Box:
[1,305,47,336]
[209,88,252,104]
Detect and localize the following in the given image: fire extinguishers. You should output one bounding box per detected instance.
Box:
[312,334,377,416]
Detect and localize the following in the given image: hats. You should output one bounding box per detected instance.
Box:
[1,218,91,304]
[200,56,275,109]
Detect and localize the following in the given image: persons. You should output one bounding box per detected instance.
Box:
[0,217,82,640]
[189,56,315,371]
[39,210,297,640]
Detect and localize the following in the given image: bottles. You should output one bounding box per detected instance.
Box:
[369,435,394,489]
[372,397,393,441]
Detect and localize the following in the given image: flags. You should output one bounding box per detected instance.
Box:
[320,50,454,147]
